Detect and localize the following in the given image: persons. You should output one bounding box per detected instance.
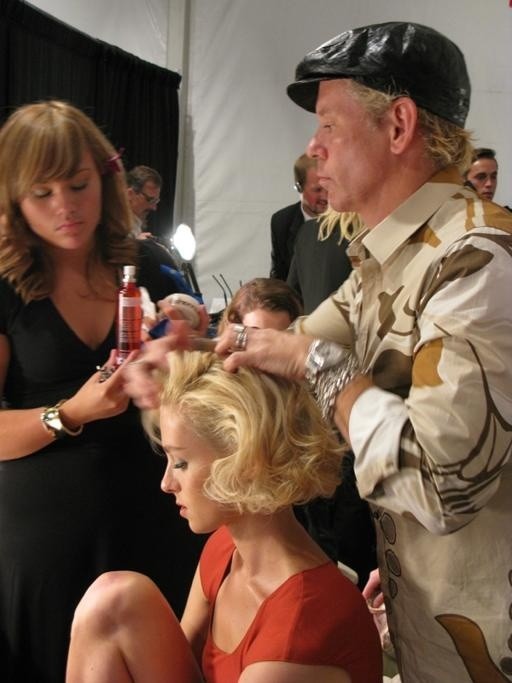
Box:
[65,356,384,682]
[213,24,512,683]
[361,568,385,608]
[0,102,216,683]
[122,164,164,236]
[218,280,301,352]
[268,152,329,289]
[285,193,375,589]
[465,147,499,204]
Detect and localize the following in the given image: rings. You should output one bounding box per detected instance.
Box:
[233,324,247,332]
[235,333,247,348]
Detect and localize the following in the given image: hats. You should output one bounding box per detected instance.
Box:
[283,21,471,129]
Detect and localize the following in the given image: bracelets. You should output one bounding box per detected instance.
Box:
[313,354,361,445]
[41,398,84,440]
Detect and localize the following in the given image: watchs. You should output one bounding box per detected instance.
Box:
[303,339,351,400]
